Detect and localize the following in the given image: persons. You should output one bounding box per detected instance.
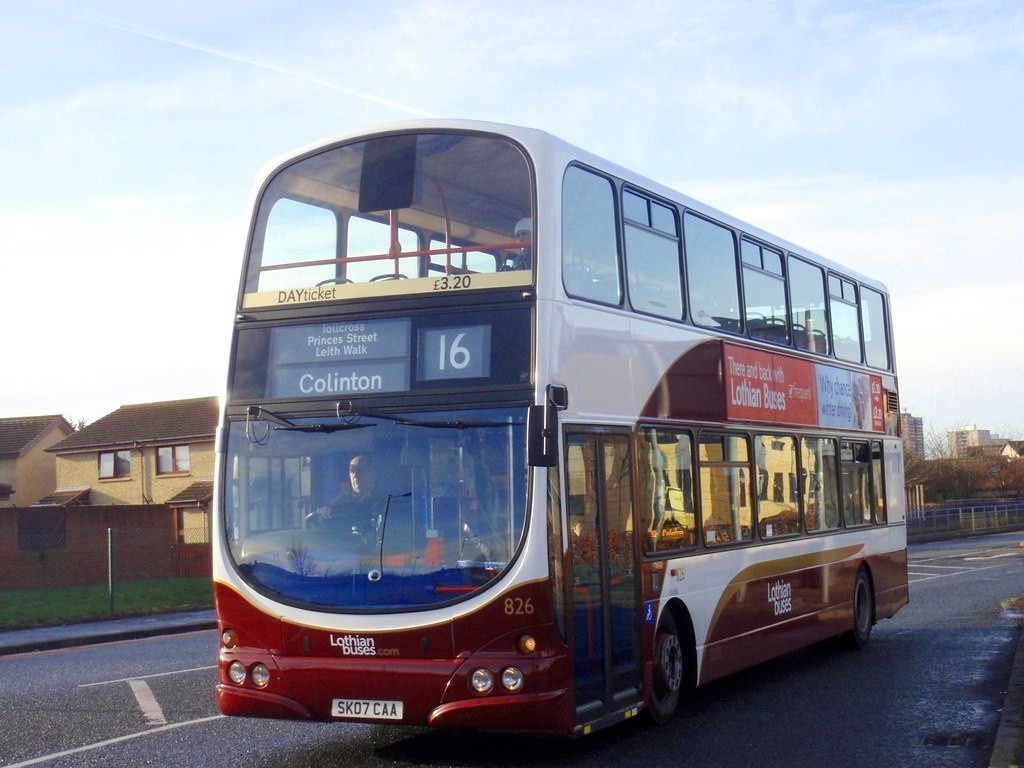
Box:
[826,493,855,528]
[511,217,532,270]
[316,455,411,554]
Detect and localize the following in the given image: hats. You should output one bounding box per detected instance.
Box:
[515,218,531,234]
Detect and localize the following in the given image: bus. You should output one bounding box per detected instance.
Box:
[208,120,909,742]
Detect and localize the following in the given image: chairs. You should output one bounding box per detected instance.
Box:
[745,311,830,355]
